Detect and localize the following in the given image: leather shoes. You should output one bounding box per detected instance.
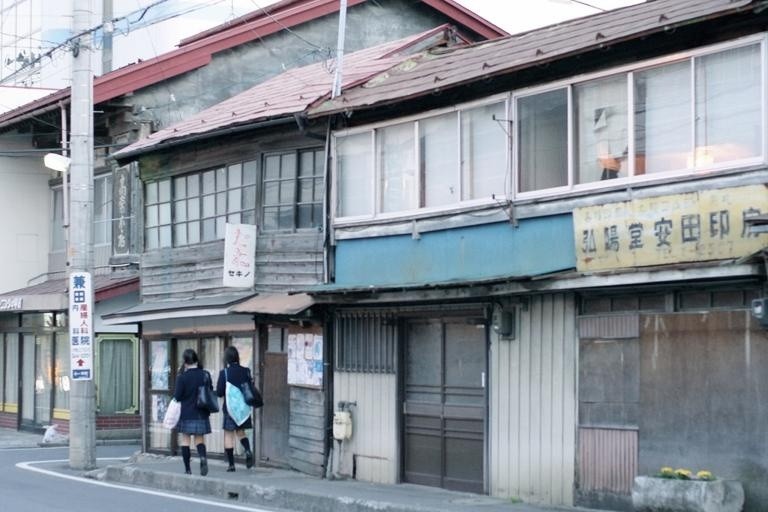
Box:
[226,466,235,472]
[246,453,252,468]
[201,466,209,476]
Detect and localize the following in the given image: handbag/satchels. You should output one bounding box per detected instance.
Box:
[164,398,181,429]
[197,386,219,412]
[241,380,264,407]
[226,382,252,425]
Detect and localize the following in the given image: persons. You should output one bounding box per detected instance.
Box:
[215,346,253,472]
[173,349,213,476]
